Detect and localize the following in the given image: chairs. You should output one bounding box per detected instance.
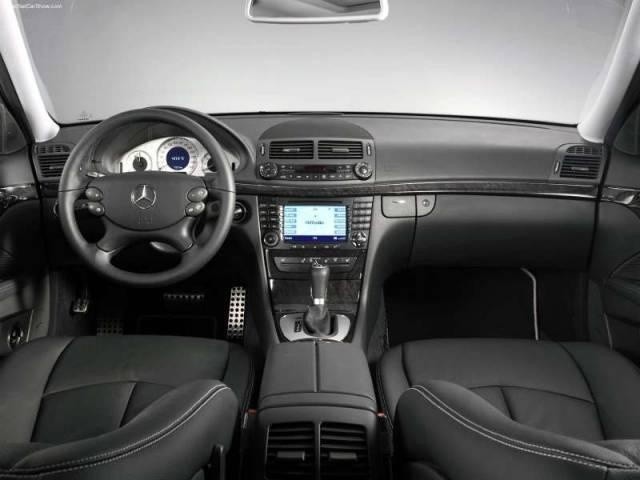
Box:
[380,338,640,480]
[1,332,255,479]
